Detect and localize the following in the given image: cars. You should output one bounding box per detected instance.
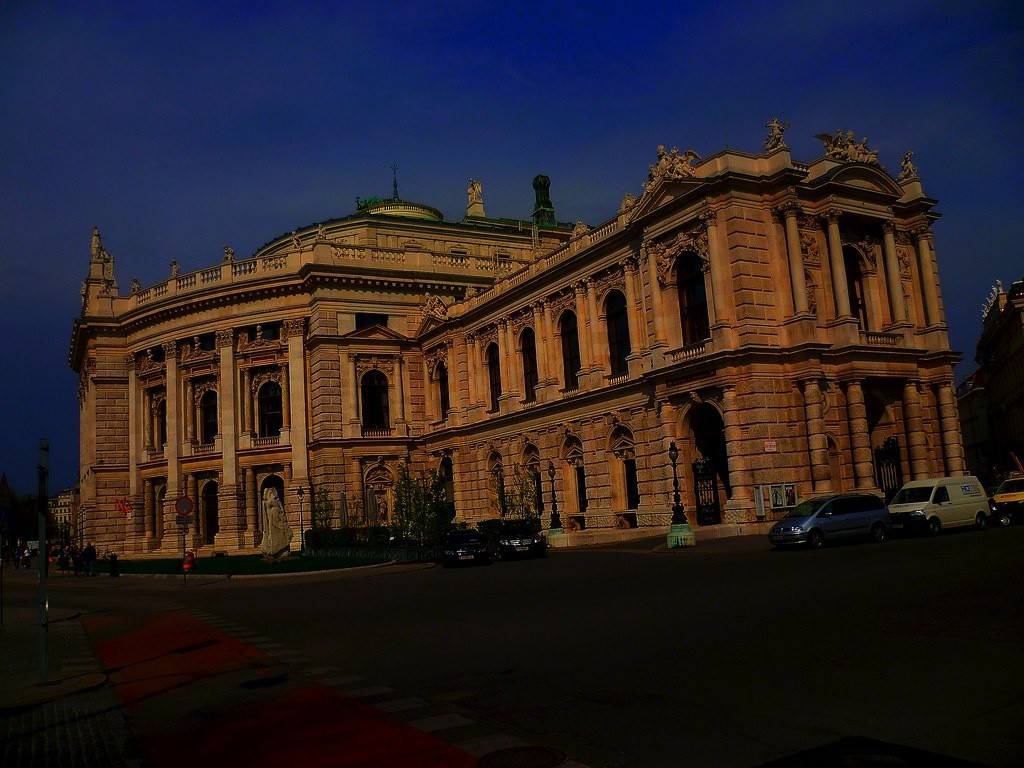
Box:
[497,518,547,560]
[441,529,489,569]
[769,494,892,548]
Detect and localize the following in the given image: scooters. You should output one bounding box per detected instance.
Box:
[988,498,1011,527]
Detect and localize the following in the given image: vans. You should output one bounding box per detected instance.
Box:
[886,476,992,536]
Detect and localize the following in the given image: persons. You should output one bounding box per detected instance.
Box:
[290,230,302,249]
[169,259,181,276]
[222,244,235,262]
[314,224,327,242]
[376,494,386,521]
[642,144,701,199]
[822,130,920,183]
[91,224,104,260]
[2,534,97,577]
[763,117,790,154]
[130,277,141,293]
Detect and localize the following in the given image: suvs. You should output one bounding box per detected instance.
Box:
[991,472,1024,517]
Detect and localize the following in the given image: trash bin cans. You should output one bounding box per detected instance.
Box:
[183,552,194,575]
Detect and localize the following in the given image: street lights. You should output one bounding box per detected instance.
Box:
[297,486,306,557]
[668,440,696,549]
